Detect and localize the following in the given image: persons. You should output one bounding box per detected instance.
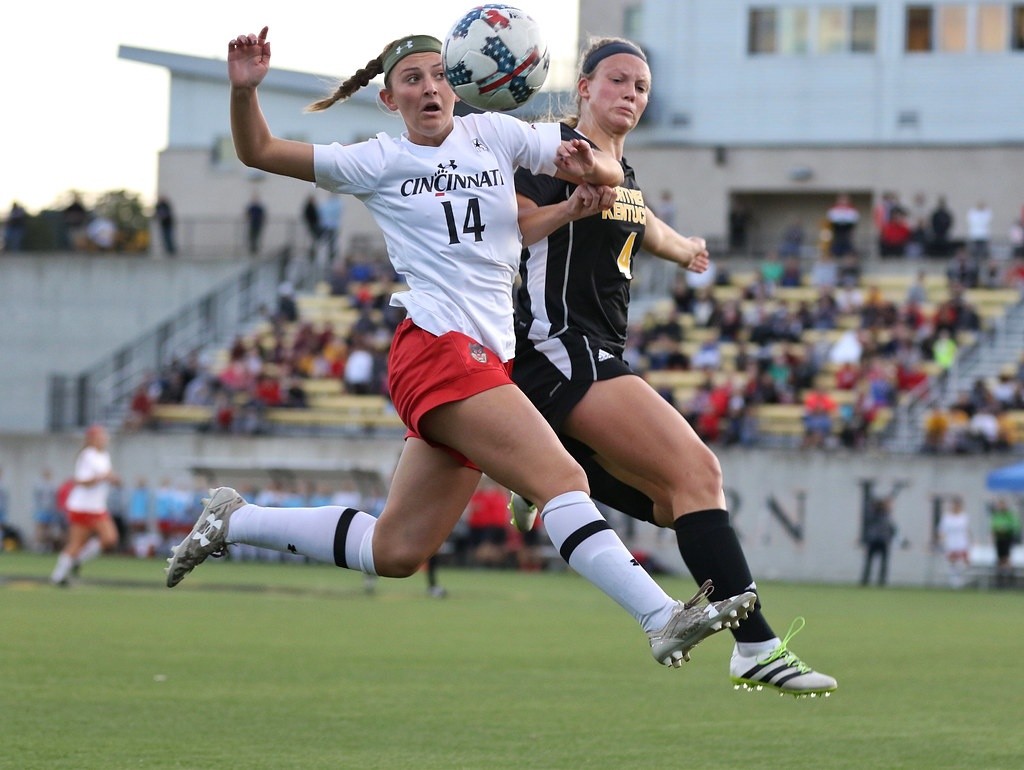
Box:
[861,497,896,587]
[515,36,838,699]
[622,183,1024,453]
[164,27,758,668]
[51,426,120,584]
[937,495,971,571]
[989,496,1020,566]
[63,202,119,251]
[246,195,265,252]
[131,234,408,434]
[154,192,177,253]
[9,203,26,220]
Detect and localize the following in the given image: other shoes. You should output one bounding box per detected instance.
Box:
[71,554,83,580]
[365,574,377,593]
[56,578,73,589]
[426,584,448,598]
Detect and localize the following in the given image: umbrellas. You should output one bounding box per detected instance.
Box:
[985,462,1024,491]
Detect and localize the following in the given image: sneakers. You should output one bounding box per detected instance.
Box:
[647,579,757,668]
[729,616,837,699]
[163,486,249,588]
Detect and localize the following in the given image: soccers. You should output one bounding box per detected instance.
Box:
[440,2,549,113]
